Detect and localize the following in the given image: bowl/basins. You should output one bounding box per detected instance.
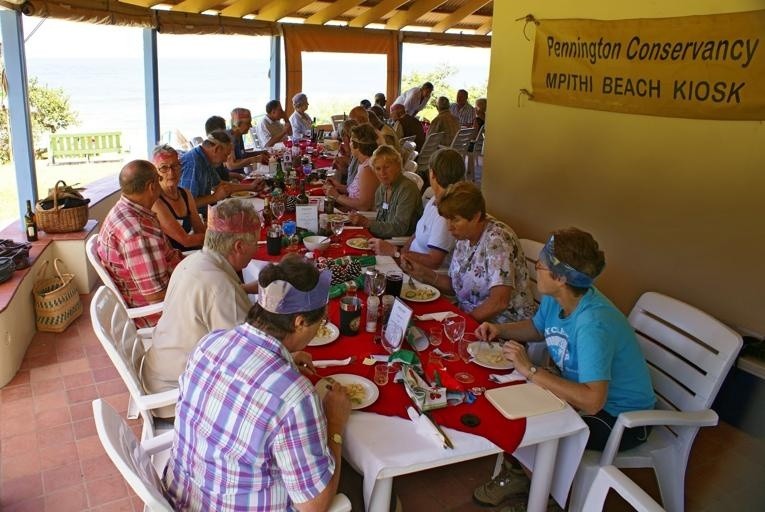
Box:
[303,235,332,253]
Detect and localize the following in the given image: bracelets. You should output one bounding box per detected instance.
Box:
[431,270,439,286]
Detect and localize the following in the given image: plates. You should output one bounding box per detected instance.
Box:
[467,340,516,371]
[307,320,340,347]
[230,190,259,199]
[314,372,381,412]
[401,282,440,302]
[299,153,340,199]
[347,237,373,252]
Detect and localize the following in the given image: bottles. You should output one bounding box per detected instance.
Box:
[262,157,309,221]
[22,199,39,242]
[311,117,319,140]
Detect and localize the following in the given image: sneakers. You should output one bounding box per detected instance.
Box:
[472,463,530,506]
[501,501,563,512]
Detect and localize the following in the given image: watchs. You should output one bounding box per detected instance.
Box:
[394,245,402,261]
[327,432,344,446]
[527,364,537,381]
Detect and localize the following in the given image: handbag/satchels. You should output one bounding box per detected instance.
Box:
[0,256,16,283]
[32,260,83,333]
[0,238,32,270]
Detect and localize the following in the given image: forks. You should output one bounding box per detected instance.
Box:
[404,265,418,289]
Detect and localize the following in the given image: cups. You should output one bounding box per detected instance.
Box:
[266,228,284,256]
[340,270,403,336]
[374,364,388,386]
[430,328,444,344]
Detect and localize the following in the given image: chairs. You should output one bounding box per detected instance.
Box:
[85,114,745,512]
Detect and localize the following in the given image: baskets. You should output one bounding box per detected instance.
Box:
[34,181,89,233]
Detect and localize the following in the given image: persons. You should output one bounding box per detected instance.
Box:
[142,199,259,423]
[160,251,404,512]
[366,147,466,305]
[149,145,205,252]
[348,144,425,241]
[472,228,658,512]
[402,182,536,347]
[96,158,186,329]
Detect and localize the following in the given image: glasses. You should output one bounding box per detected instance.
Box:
[535,259,552,272]
[157,162,180,173]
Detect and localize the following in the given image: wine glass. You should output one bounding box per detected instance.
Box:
[271,200,297,251]
[441,314,466,361]
[453,332,481,384]
[330,217,344,249]
[380,324,404,375]
